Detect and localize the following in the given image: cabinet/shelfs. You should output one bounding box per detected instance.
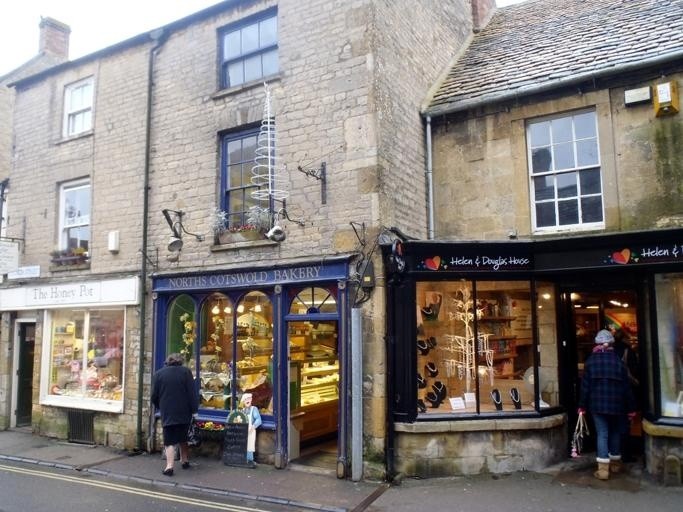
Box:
[204,301,340,443]
[459,288,518,381]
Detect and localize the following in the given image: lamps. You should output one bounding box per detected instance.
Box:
[262,207,307,244]
[167,221,206,252]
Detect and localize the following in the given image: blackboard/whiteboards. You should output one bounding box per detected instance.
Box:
[223,423,248,464]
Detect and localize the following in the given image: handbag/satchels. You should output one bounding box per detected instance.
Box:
[570,412,596,458]
[186,417,203,449]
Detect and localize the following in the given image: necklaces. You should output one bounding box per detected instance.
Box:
[416,339,428,350]
[508,388,519,403]
[418,403,427,411]
[432,382,444,392]
[424,390,437,402]
[420,306,432,314]
[417,376,425,383]
[490,390,501,404]
[427,337,437,348]
[425,362,438,373]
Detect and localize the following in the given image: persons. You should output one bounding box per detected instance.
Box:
[427,291,442,321]
[149,352,200,475]
[576,328,638,482]
[239,393,262,467]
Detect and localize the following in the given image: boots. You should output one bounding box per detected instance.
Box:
[593,457,610,480]
[608,453,623,473]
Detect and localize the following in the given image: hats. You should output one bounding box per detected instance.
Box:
[241,393,252,401]
[595,329,615,344]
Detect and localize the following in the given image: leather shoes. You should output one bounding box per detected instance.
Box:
[162,468,173,476]
[181,462,190,469]
